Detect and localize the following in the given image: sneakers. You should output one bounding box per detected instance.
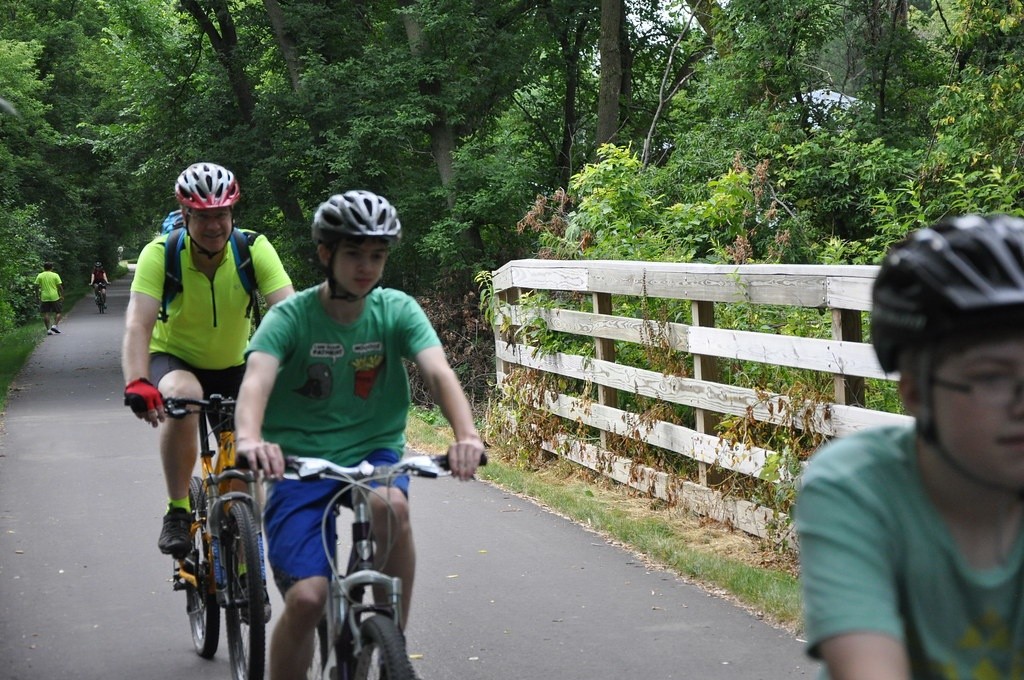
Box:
[232,572,272,626]
[870,212,1024,371]
[157,507,193,556]
[51,325,61,333]
[47,329,55,335]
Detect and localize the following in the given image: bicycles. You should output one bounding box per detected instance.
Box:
[144,394,270,680]
[205,453,487,680]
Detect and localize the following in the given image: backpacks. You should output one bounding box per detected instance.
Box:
[159,210,259,325]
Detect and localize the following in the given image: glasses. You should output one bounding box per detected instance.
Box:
[921,374,1024,402]
[186,210,230,221]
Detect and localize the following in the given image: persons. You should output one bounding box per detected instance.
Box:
[235,188,483,680]
[793,214,1024,680]
[34,262,63,335]
[89,261,110,309]
[120,162,485,680]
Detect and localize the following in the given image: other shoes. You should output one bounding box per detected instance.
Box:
[95,296,98,301]
[103,303,108,309]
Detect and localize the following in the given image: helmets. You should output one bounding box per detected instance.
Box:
[175,161,241,211]
[313,189,402,268]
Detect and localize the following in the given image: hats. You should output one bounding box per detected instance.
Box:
[94,261,102,267]
